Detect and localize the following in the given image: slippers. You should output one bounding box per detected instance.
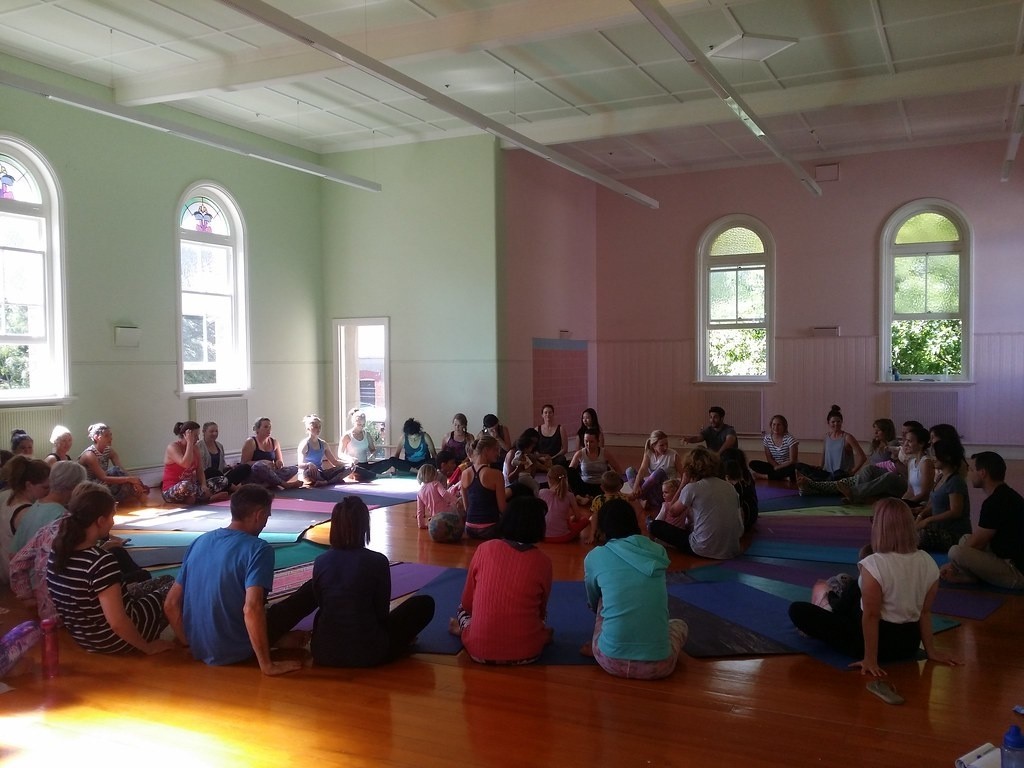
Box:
[866,678,905,705]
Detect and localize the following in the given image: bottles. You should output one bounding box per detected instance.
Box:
[39,620,59,679]
[1001,725,1024,767]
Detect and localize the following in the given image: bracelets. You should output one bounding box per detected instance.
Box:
[918,514,922,518]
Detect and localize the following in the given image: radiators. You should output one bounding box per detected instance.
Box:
[0,405,63,467]
[891,390,959,438]
[191,397,249,454]
[703,389,763,435]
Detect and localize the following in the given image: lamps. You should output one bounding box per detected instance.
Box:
[1,0,1023,210]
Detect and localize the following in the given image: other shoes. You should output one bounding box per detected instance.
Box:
[795,469,808,497]
[835,481,852,506]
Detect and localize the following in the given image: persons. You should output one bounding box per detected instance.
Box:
[46,490,177,656]
[0,403,1023,679]
[530,405,568,472]
[164,483,318,675]
[575,408,605,452]
[647,445,744,560]
[788,496,965,677]
[460,435,508,540]
[578,497,689,679]
[310,495,435,669]
[749,414,800,482]
[448,481,556,667]
[297,413,357,487]
[566,428,625,506]
[76,423,144,500]
[914,438,973,554]
[161,421,228,505]
